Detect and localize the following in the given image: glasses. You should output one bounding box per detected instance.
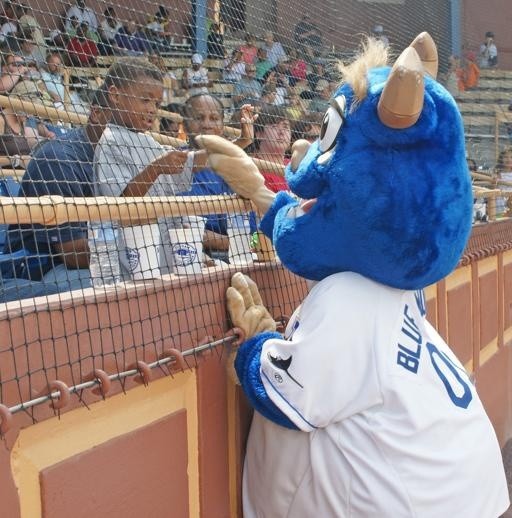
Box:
[5,61,26,67]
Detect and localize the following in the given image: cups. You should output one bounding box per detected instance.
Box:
[120,223,162,280]
[470,202,487,223]
[168,229,204,275]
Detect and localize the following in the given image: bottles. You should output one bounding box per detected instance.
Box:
[224,213,256,268]
[87,221,124,292]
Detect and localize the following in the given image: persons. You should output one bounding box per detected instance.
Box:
[0,1,511,300]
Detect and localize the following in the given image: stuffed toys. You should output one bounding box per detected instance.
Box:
[192,28,509,518]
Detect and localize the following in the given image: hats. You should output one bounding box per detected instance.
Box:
[314,78,329,92]
[191,53,203,65]
